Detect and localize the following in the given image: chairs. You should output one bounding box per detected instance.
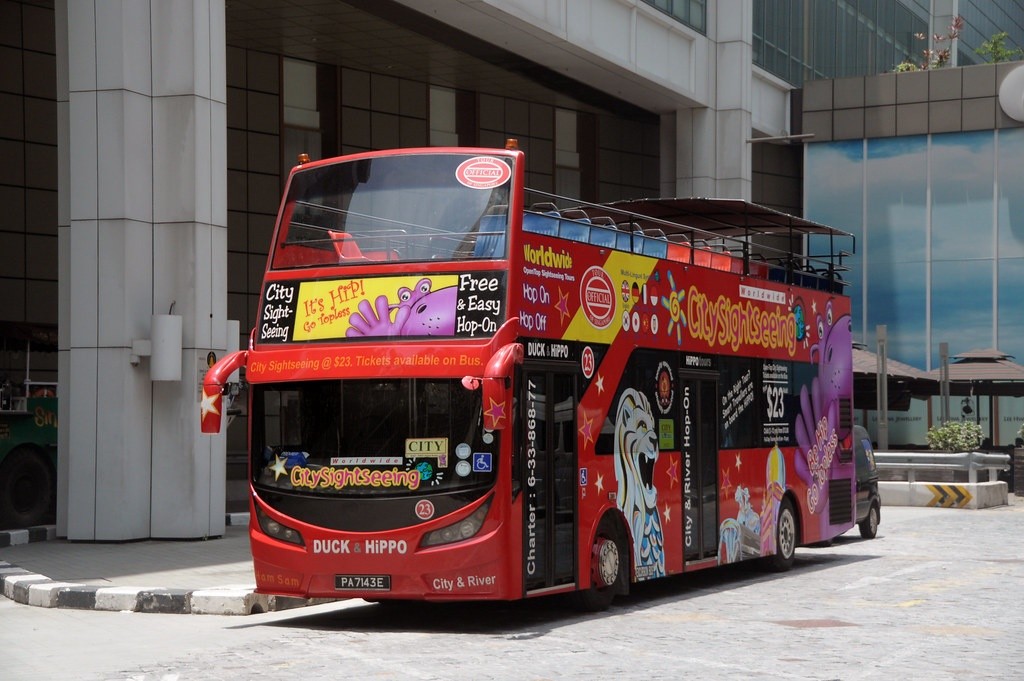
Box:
[331,200,841,296]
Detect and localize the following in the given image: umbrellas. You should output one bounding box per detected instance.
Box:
[852,341,1024,445]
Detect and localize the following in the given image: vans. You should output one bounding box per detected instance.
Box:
[811,423,882,547]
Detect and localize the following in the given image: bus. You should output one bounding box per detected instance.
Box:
[200,136,858,613]
